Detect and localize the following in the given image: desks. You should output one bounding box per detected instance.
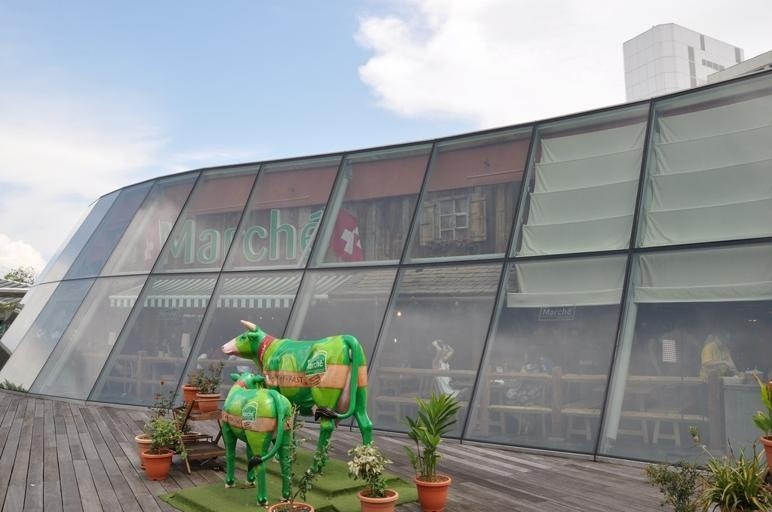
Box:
[375,365,554,439]
[560,372,706,446]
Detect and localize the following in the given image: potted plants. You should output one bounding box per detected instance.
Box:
[347,441,399,512]
[750,371,772,474]
[196,360,224,413]
[403,388,463,512]
[134,433,156,469]
[689,425,772,512]
[268,400,333,512]
[181,367,204,410]
[141,415,188,480]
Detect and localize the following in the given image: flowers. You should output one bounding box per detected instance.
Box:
[149,380,176,421]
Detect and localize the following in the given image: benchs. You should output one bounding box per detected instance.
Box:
[107,376,232,400]
[561,405,710,448]
[373,395,553,441]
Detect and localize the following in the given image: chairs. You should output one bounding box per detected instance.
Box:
[116,352,256,397]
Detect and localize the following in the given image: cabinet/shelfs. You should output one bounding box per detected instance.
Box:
[721,382,771,466]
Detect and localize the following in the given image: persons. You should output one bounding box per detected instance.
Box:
[643,331,670,374]
[699,332,736,377]
[430,340,471,400]
[228,355,250,374]
[505,344,547,436]
